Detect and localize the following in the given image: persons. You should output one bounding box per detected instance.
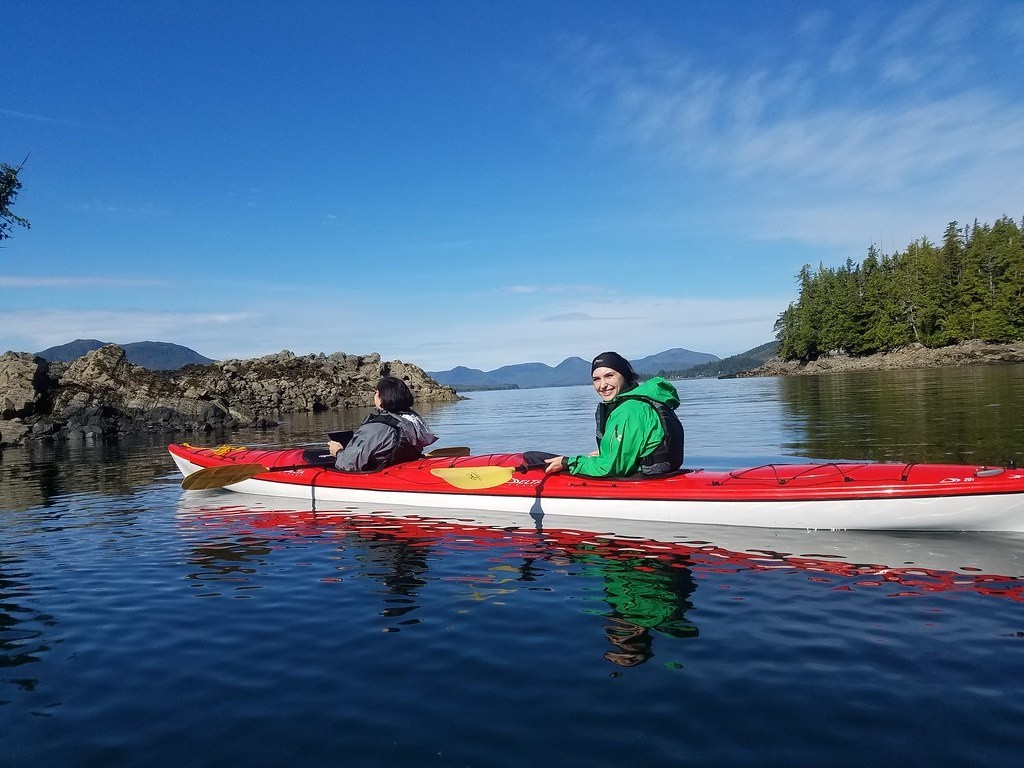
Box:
[327,376,439,474]
[545,351,685,478]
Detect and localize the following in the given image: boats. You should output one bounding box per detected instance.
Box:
[166,439,1022,529]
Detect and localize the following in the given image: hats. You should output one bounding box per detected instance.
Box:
[592,352,634,385]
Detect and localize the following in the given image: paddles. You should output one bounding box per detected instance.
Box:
[180,446,552,491]
